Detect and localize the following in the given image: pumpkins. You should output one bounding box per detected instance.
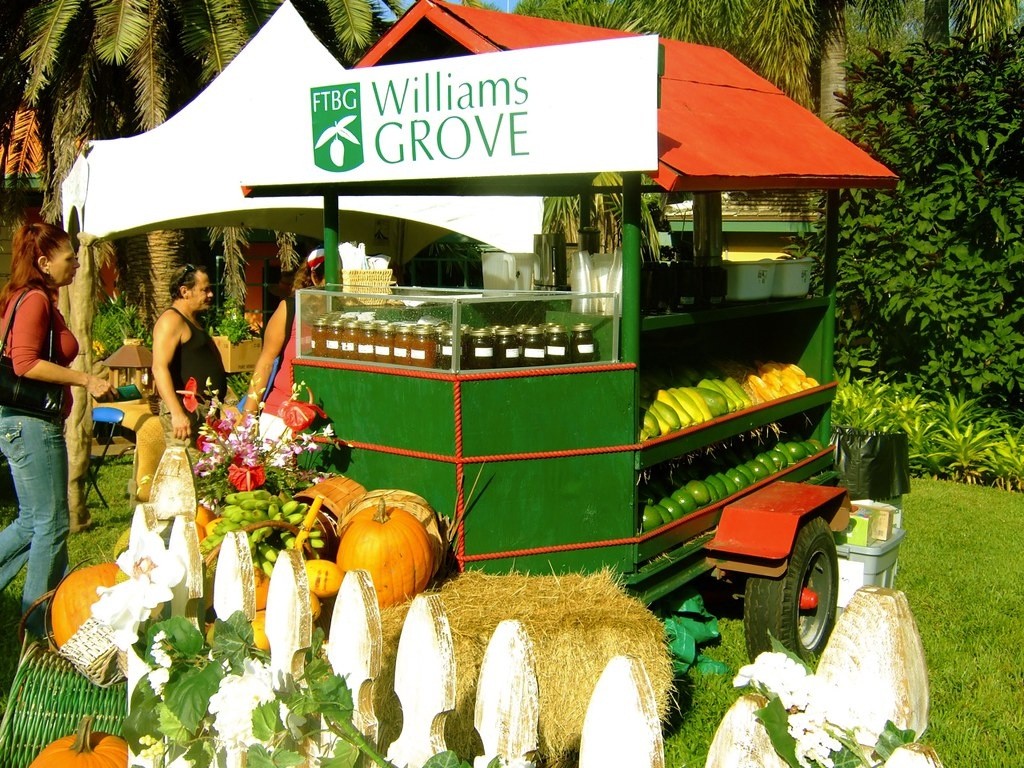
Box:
[336,497,434,609]
[28,716,128,768]
[51,562,120,650]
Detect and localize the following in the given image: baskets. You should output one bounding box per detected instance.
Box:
[0,628,130,768]
[43,556,145,687]
[339,268,393,306]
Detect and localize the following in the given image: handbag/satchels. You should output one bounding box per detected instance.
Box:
[0,279,65,426]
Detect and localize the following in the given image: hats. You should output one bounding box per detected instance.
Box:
[308,248,324,270]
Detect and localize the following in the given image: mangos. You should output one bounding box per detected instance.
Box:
[638,432,822,533]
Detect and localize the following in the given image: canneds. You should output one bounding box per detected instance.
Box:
[311,311,453,370]
[460,322,596,370]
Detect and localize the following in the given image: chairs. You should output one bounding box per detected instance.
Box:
[83,406,126,510]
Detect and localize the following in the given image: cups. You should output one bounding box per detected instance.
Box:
[571,248,623,318]
[533,233,567,287]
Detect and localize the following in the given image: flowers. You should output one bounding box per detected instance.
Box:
[175,370,357,520]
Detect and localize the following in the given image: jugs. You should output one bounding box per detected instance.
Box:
[510,253,541,292]
[481,252,517,292]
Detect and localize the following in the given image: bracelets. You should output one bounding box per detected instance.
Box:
[243,409,259,413]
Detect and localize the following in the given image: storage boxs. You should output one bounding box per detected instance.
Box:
[211,334,264,374]
[833,503,880,547]
[869,506,895,540]
[832,526,909,608]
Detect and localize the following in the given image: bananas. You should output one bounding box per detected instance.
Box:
[201,489,325,577]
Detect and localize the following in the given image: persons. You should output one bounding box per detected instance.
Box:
[218,247,326,469]
[152,263,227,479]
[0,222,119,643]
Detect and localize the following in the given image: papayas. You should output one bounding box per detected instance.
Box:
[637,367,752,442]
[114,506,346,651]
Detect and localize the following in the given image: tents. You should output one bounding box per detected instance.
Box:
[59,0,547,535]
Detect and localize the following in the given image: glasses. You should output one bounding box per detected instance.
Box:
[178,264,198,286]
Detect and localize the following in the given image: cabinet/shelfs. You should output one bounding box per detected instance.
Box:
[634,294,842,564]
